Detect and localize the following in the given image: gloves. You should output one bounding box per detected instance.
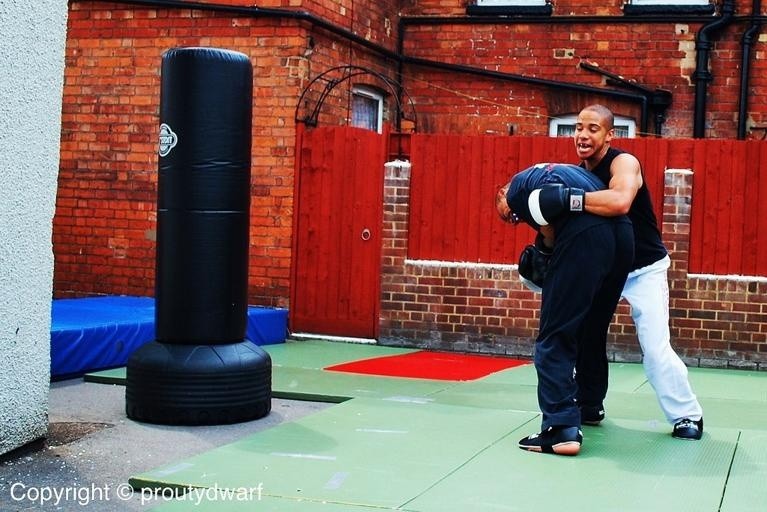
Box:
[519,233,552,292]
[526,183,585,226]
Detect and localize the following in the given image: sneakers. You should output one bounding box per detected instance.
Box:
[672,417,703,440]
[519,405,605,455]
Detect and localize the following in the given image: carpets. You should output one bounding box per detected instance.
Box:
[322,348,535,386]
